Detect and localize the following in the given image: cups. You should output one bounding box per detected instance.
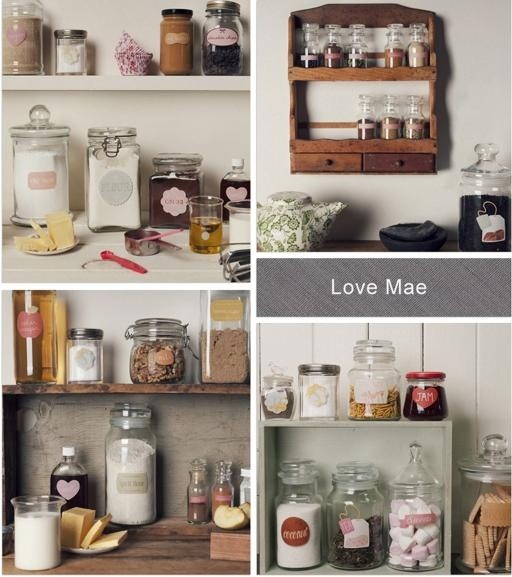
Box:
[10,494,65,569]
[188,196,223,253]
[224,198,251,251]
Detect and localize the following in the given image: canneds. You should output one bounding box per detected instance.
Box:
[260,372,297,420]
[159,9,194,75]
[403,371,449,421]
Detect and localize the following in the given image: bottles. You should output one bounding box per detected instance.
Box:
[383,24,404,68]
[262,339,509,573]
[9,104,72,224]
[86,126,144,235]
[219,158,250,223]
[54,29,88,75]
[298,22,321,67]
[345,23,369,69]
[3,0,45,76]
[400,94,426,141]
[355,94,377,140]
[458,142,511,252]
[15,290,251,531]
[406,23,429,69]
[376,95,405,140]
[322,22,344,68]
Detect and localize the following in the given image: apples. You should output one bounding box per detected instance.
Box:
[213,502,250,529]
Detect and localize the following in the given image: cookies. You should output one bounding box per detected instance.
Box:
[462,493,511,575]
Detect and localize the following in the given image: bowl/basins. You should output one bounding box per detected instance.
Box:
[379,223,448,251]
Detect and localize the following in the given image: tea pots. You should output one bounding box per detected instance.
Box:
[256,191,347,252]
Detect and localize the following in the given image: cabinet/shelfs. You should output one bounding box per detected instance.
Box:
[3,72,251,283]
[1,383,252,575]
[256,416,453,575]
[286,4,440,173]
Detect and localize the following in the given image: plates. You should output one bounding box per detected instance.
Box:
[63,548,119,555]
[23,233,81,255]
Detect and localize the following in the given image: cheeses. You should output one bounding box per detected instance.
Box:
[60,506,128,550]
